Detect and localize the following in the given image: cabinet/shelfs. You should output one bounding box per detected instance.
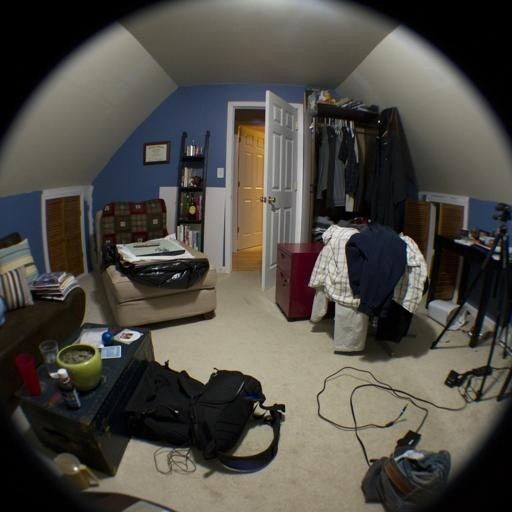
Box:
[274,240,320,321]
[176,130,212,253]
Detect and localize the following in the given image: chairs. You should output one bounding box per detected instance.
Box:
[93,198,217,328]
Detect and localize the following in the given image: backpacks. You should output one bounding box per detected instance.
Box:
[123,359,285,471]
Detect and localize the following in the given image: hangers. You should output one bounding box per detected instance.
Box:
[317,116,356,137]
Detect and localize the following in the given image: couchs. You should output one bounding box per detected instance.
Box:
[0,231,86,436]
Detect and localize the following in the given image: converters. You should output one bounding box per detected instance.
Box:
[472,365,492,377]
[445,370,458,388]
[397,430,421,448]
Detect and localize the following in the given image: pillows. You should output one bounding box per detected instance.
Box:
[0,237,41,326]
[102,198,167,265]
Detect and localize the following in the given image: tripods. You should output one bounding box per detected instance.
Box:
[430,226,512,402]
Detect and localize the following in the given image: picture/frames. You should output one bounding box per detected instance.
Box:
[143,141,169,166]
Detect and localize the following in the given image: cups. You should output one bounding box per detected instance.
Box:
[39,338,59,365]
[53,452,101,495]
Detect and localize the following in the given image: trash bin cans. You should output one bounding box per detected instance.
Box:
[427,299,467,331]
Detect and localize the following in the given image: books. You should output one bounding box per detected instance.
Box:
[30,269,81,301]
[176,223,200,253]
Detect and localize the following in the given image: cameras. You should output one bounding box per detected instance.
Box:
[492,203,512,226]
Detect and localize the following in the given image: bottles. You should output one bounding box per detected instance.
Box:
[57,368,82,410]
[179,193,202,222]
[187,138,202,156]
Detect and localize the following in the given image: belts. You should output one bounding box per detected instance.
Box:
[384,462,415,496]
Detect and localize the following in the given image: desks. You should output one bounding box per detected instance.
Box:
[15,322,157,476]
[432,230,511,349]
